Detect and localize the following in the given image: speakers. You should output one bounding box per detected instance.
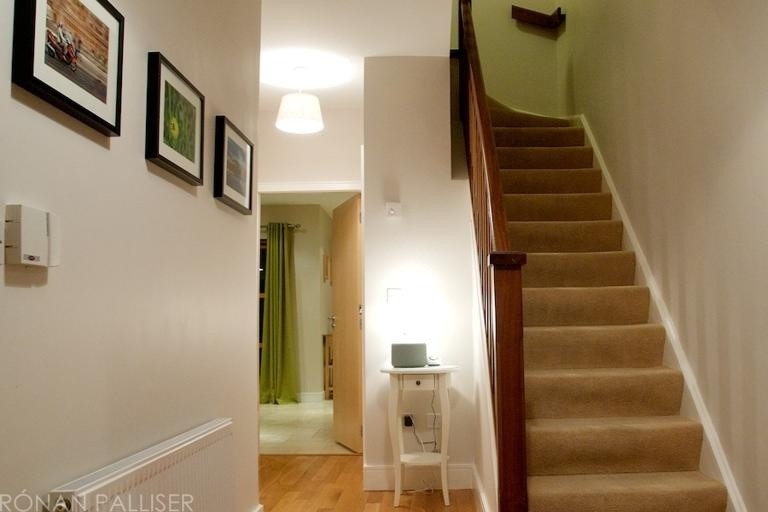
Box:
[392,343,426,367]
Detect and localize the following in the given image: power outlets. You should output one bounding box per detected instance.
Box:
[400,413,415,429]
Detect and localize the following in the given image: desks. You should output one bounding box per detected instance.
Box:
[378,364,458,507]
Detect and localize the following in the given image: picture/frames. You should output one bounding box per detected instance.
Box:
[143,50,206,186]
[323,255,329,278]
[9,0,126,138]
[212,115,253,216]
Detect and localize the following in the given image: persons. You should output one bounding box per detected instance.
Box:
[55,22,72,55]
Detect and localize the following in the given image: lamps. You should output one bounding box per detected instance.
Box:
[274,90,324,134]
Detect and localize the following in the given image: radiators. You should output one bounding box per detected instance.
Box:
[45,415,238,512]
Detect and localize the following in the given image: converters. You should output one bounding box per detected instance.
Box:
[404,416,413,427]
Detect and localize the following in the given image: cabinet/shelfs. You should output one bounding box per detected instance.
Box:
[322,333,333,401]
